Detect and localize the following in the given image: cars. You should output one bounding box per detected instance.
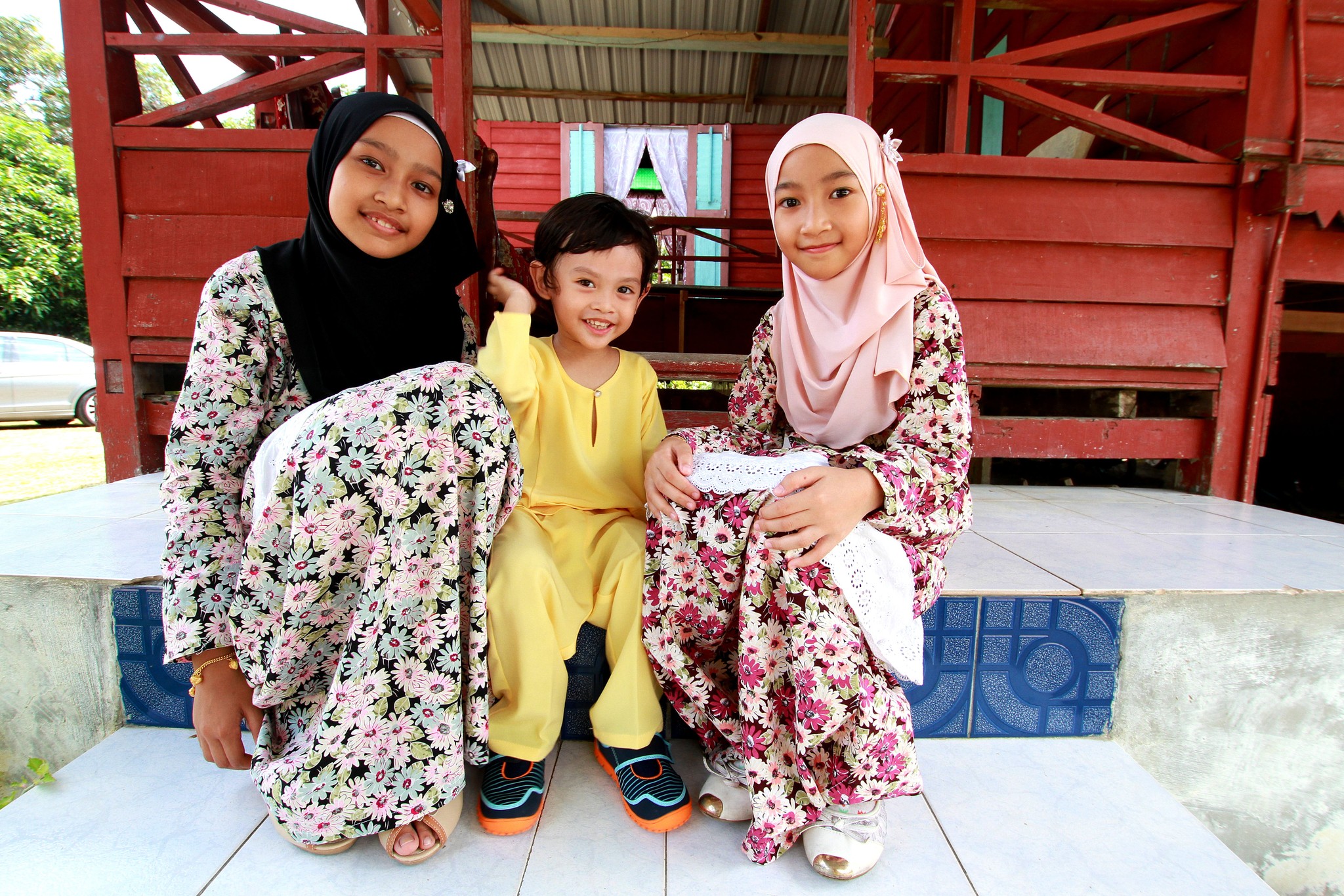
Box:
[0,332,96,426]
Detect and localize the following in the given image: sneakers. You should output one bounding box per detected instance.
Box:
[475,748,545,835]
[594,731,693,833]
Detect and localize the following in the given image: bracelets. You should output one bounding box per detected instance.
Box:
[188,652,239,699]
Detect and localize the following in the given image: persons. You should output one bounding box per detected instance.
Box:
[474,192,693,836]
[641,111,972,881]
[161,90,525,866]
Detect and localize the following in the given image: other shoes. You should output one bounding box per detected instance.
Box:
[803,799,886,879]
[698,763,754,823]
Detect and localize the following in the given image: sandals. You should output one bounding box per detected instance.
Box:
[268,806,358,855]
[377,787,463,866]
